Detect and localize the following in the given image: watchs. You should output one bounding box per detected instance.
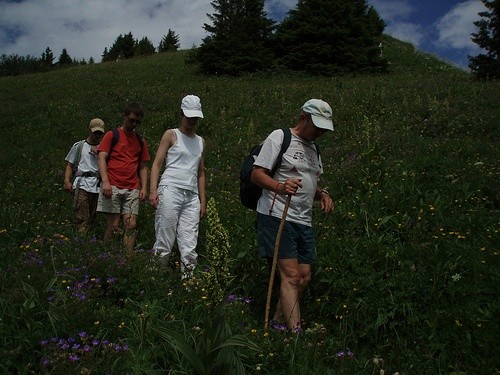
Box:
[320,190,330,194]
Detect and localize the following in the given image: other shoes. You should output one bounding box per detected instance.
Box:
[269,320,288,331]
[290,327,303,332]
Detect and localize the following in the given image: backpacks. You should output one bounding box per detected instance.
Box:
[239,128,321,211]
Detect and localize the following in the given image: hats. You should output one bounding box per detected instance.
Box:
[181,94,204,118]
[89,117,105,134]
[301,98,334,131]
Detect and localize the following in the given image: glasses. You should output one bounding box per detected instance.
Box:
[126,115,142,125]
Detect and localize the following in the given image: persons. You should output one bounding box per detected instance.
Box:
[63,117,102,237]
[98,103,149,259]
[149,95,207,293]
[239,99,335,334]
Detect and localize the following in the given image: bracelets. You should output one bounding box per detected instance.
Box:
[276,183,282,195]
